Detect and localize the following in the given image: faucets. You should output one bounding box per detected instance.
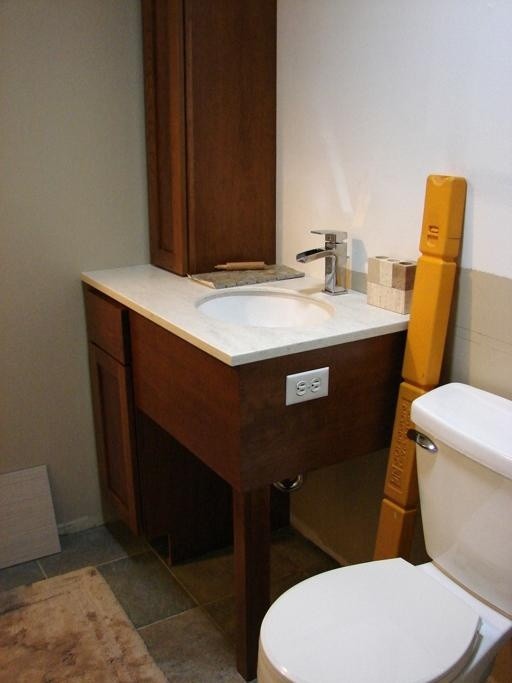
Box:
[297,225,350,293]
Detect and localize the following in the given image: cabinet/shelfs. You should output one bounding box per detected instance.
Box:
[139,3,278,277]
[81,284,290,566]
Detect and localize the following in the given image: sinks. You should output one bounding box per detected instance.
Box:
[192,283,338,333]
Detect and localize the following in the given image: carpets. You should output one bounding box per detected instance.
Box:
[0,567,169,683]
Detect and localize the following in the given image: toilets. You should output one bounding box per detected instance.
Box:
[256,380,511,682]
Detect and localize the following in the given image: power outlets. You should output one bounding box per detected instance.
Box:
[284,366,330,406]
